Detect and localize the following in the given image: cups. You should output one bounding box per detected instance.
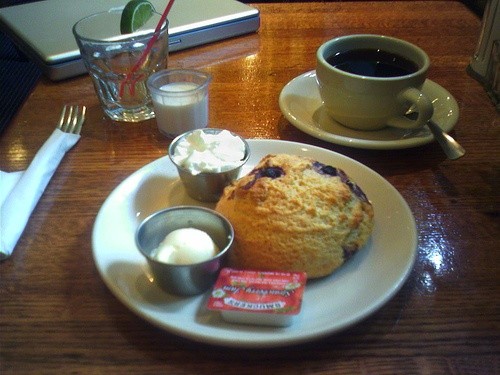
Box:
[168,125,250,201]
[73,7,169,120]
[145,68,211,134]
[135,205,234,295]
[315,34,433,131]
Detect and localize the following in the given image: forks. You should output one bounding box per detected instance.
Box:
[58,103,88,134]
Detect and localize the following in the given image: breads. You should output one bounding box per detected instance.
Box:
[216,154,375,277]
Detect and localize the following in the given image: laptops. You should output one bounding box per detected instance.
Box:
[0,0,260,80]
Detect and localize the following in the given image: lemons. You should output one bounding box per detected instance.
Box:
[120,0,156,34]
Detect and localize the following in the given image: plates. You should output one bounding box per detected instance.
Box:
[93,140,418,344]
[279,69,460,149]
